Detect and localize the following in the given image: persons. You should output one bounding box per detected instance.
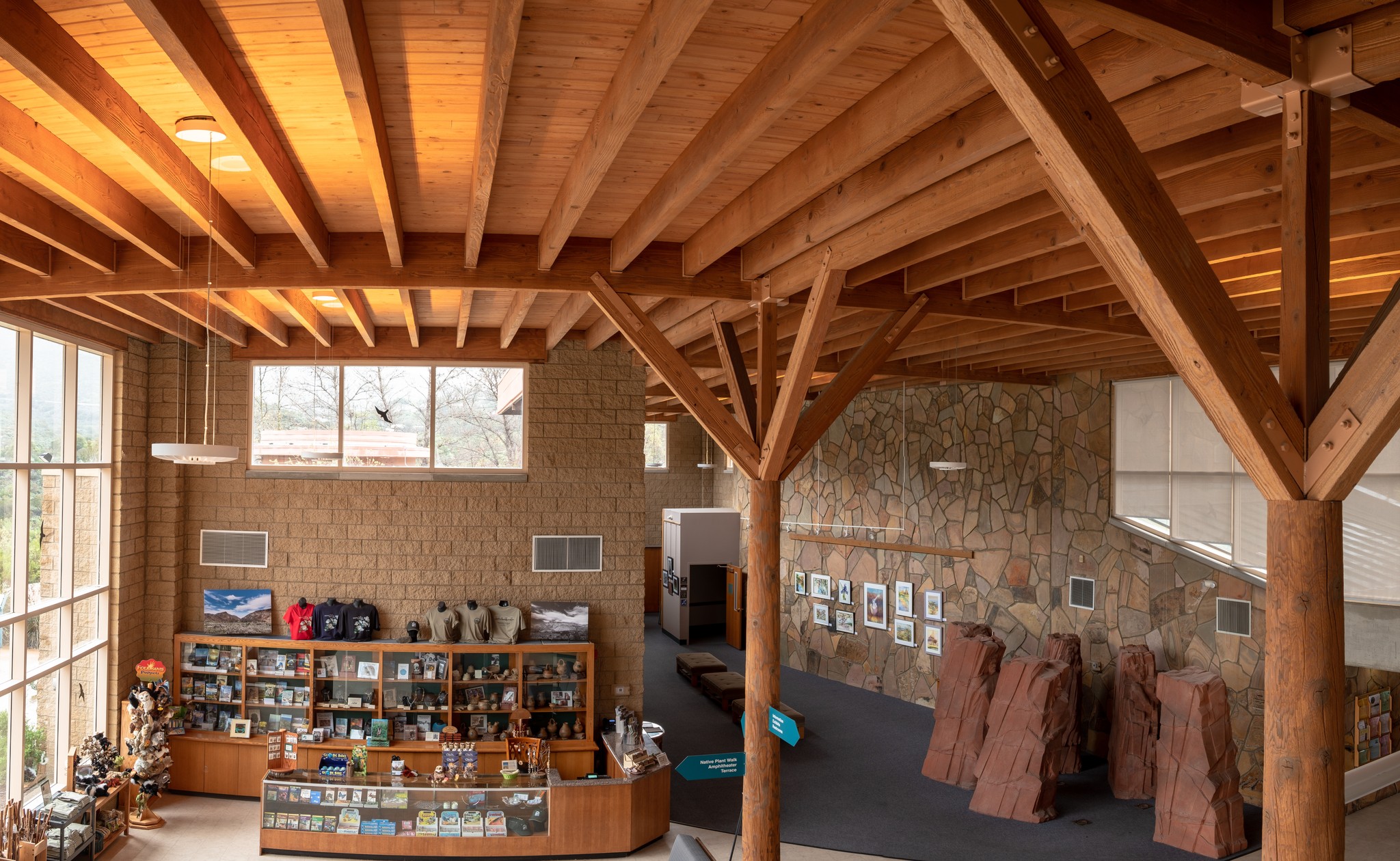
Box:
[419,721,424,732]
[423,600,526,645]
[283,597,381,642]
[435,736,437,739]
[424,721,429,732]
[435,734,436,739]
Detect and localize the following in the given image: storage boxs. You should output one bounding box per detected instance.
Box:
[263,807,508,838]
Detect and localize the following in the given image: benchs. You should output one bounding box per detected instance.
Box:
[695,670,749,710]
[730,695,806,739]
[674,650,730,677]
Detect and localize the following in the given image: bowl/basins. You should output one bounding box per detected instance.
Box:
[626,769,645,775]
[477,665,518,681]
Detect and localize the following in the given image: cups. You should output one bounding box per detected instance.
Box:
[557,659,567,675]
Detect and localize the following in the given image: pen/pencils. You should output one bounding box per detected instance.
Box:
[346,761,354,767]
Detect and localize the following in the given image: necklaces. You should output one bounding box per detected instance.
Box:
[439,692,448,701]
[407,697,416,706]
[415,687,425,702]
[322,688,346,702]
[426,695,436,705]
[364,688,374,702]
[526,746,536,776]
[547,746,551,774]
[398,692,408,704]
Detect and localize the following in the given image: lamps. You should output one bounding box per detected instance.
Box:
[923,453,978,475]
[145,111,246,470]
[688,457,719,474]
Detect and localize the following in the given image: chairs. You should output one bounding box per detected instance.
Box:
[505,737,542,766]
[667,831,720,861]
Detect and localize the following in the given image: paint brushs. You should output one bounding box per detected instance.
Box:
[535,787,546,799]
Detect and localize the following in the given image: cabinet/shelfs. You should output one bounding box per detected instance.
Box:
[27,790,97,861]
[66,769,68,769]
[118,696,163,812]
[167,626,604,804]
[19,834,48,861]
[257,762,555,858]
[70,769,132,861]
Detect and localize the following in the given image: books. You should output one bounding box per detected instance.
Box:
[246,647,310,734]
[314,653,451,680]
[182,643,242,731]
[315,712,431,749]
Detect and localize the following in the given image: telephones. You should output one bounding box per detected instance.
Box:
[603,718,616,731]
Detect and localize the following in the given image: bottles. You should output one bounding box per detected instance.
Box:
[467,721,515,740]
[453,664,474,681]
[537,691,546,707]
[558,701,567,705]
[467,703,475,711]
[527,659,537,674]
[478,693,518,711]
[526,696,535,711]
[538,728,548,740]
[559,722,571,739]
[573,660,584,672]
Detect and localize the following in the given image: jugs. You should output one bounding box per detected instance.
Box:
[457,723,467,737]
[548,718,557,733]
[573,687,582,703]
[457,690,480,705]
[573,717,583,733]
[543,663,553,679]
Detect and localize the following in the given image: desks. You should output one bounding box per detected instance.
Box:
[637,719,667,749]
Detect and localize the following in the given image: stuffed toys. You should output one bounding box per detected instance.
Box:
[441,741,476,752]
[428,765,452,784]
[80,681,174,796]
[97,809,124,826]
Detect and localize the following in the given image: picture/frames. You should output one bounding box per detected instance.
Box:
[835,608,856,635]
[793,570,806,596]
[893,618,915,648]
[812,603,830,627]
[923,589,943,622]
[862,580,890,632]
[894,580,913,618]
[922,623,943,657]
[810,571,833,600]
[662,554,688,608]
[838,577,852,606]
